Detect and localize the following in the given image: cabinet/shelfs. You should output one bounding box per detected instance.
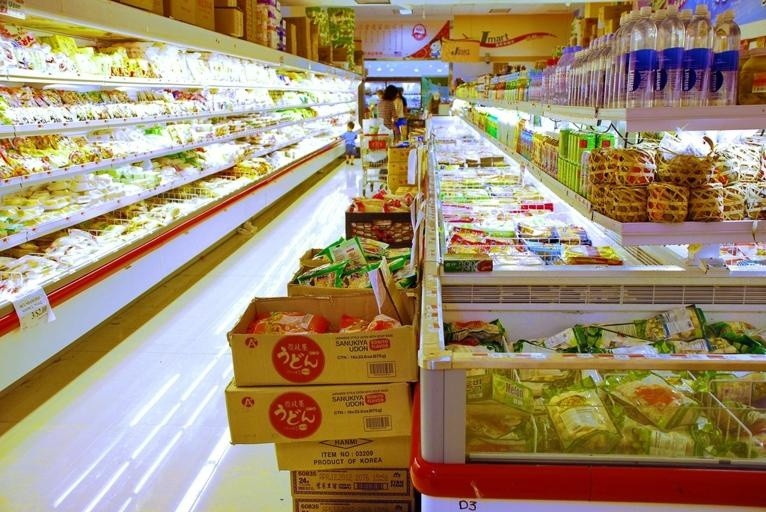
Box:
[410,97,766,512]
[0,0,362,391]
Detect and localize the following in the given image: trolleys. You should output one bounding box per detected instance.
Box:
[360,131,391,197]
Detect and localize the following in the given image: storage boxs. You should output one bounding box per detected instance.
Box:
[226,250,418,512]
[387,149,419,196]
[117,0,364,73]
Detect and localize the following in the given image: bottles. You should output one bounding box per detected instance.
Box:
[476,3,766,181]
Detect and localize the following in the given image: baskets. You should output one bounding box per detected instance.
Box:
[586,146,765,222]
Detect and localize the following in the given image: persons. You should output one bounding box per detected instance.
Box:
[393,88,407,142]
[375,90,384,101]
[377,84,400,180]
[328,120,358,165]
[399,87,408,113]
